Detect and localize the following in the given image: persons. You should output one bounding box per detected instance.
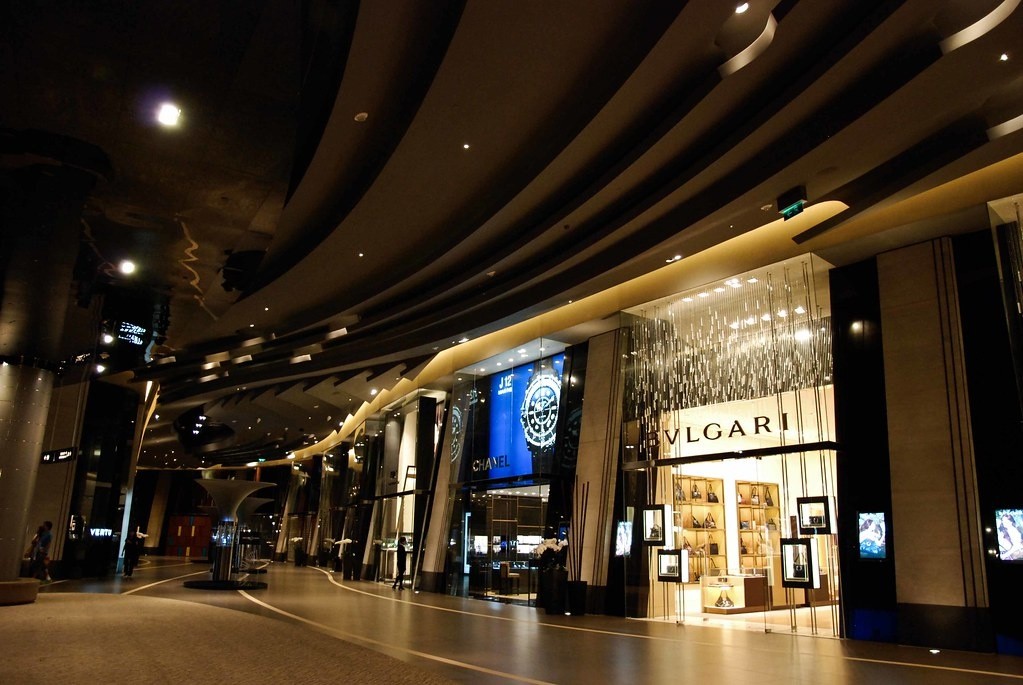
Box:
[123,528,139,578]
[29,521,53,582]
[392,537,407,590]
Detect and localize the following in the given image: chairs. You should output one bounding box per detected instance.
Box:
[500,564,521,596]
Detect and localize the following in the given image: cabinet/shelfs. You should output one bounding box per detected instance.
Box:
[736,481,780,571]
[670,474,729,584]
[700,534,836,614]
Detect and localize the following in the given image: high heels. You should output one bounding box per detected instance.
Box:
[392,584,396,589]
[398,586,404,590]
